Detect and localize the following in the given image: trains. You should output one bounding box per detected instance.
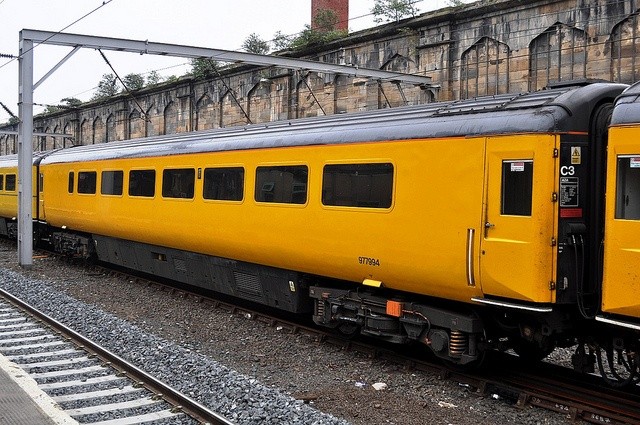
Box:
[0,78,640,376]
